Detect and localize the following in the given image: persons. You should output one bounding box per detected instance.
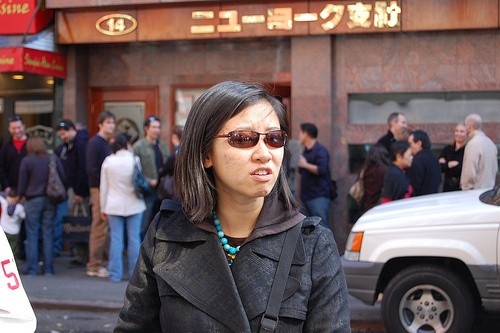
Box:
[113,80,351,333]
[16,137,66,275]
[297,122,331,226]
[0,110,469,276]
[460,114,498,191]
[100,133,146,282]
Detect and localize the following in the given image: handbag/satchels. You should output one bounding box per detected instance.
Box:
[348,166,367,202]
[46,153,67,203]
[157,174,175,200]
[62,202,91,242]
[133,153,156,195]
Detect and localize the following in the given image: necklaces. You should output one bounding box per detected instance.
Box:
[213,211,240,255]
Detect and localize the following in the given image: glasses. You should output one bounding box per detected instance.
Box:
[56,122,66,127]
[212,129,288,149]
[144,116,156,126]
[7,115,21,121]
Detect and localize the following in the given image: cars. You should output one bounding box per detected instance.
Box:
[340,171,500,333]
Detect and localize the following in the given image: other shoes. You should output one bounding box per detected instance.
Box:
[86,266,109,277]
[68,260,83,266]
